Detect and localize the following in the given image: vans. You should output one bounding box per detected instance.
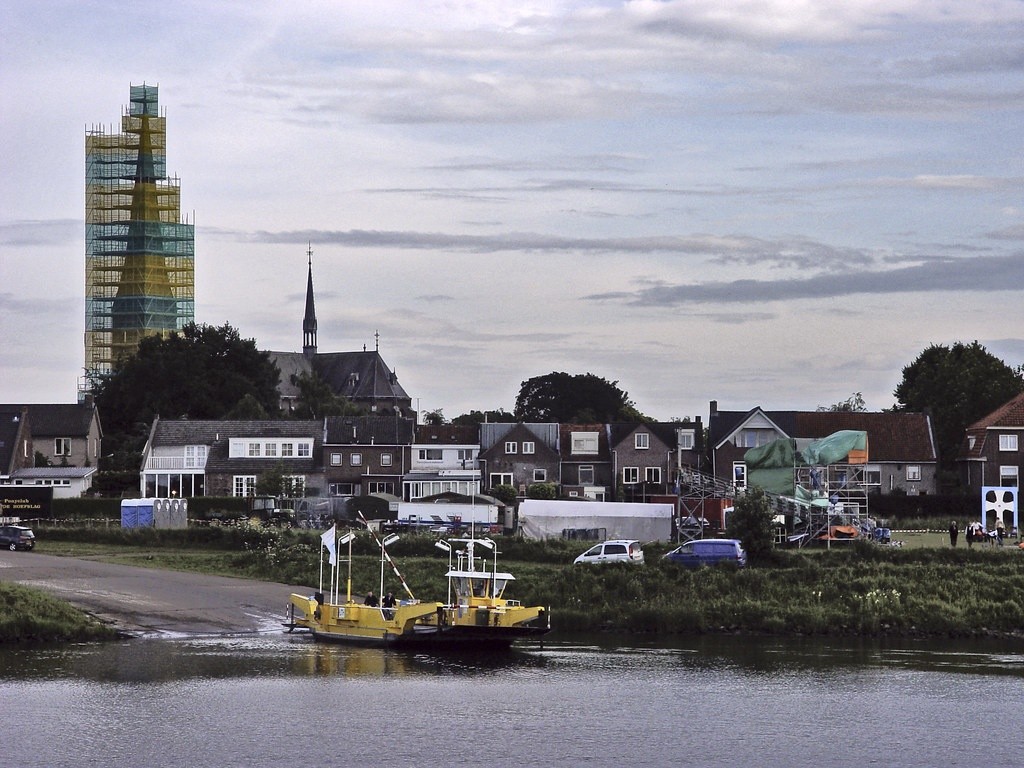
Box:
[573,540,645,565]
[661,539,746,569]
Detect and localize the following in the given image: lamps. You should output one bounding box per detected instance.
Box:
[507,600,521,608]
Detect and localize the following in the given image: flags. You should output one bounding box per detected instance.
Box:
[320,526,336,566]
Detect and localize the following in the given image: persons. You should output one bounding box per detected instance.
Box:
[383,591,396,607]
[364,592,379,607]
[964,521,975,547]
[971,520,984,531]
[867,515,876,529]
[949,521,958,547]
[995,517,1005,539]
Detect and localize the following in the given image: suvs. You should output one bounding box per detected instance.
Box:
[0,526,35,551]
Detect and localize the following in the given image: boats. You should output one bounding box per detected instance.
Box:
[281,455,551,651]
